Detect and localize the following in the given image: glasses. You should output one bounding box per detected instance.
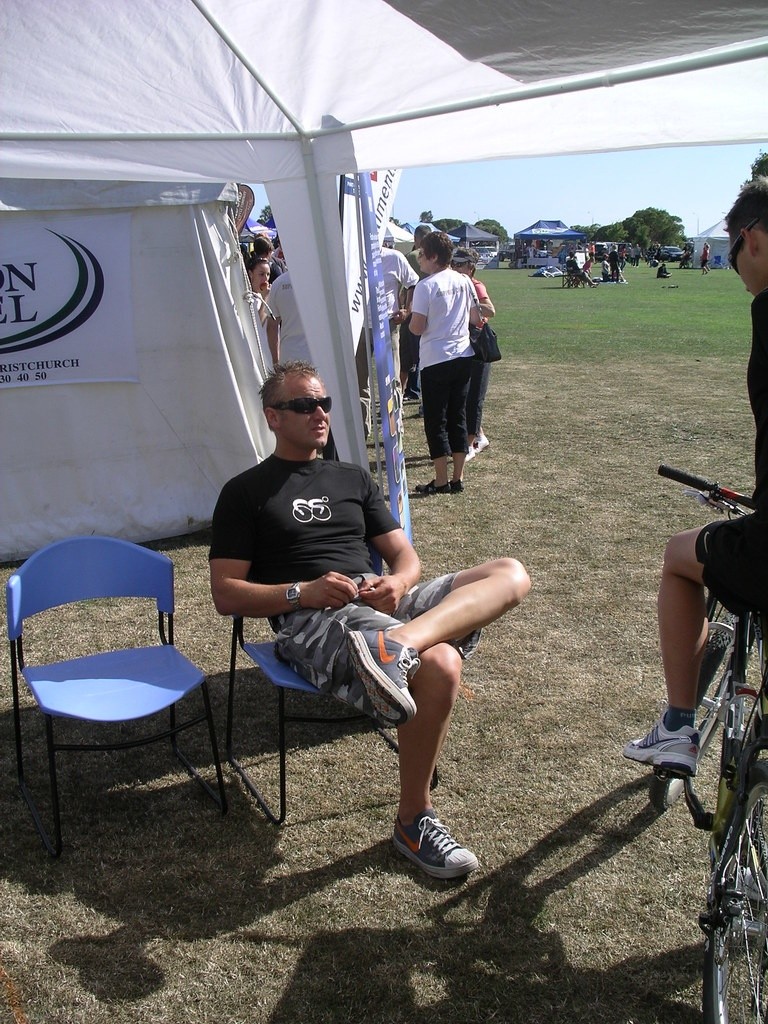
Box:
[418,251,427,258]
[728,214,763,275]
[451,261,473,268]
[270,397,332,414]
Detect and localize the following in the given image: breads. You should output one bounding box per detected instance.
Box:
[261,281,270,290]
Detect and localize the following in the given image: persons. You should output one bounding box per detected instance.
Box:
[209,361,532,880]
[559,240,710,288]
[240,233,497,496]
[623,176,768,776]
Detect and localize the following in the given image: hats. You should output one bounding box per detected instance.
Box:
[452,252,477,264]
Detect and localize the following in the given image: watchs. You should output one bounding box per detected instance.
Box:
[286,581,304,612]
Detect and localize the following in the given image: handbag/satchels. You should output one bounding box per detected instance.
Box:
[462,274,502,363]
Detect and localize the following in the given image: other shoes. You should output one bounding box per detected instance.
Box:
[465,446,475,463]
[473,435,489,453]
[419,406,423,418]
[591,283,599,288]
[403,395,411,401]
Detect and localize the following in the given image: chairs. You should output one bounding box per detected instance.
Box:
[6,533,225,859]
[555,266,588,289]
[714,256,725,269]
[225,615,442,826]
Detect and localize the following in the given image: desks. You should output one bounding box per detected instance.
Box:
[527,258,566,268]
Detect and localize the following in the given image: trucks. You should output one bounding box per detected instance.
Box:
[593,242,632,261]
[498,243,516,262]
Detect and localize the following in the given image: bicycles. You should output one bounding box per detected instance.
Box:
[650,463,767,1024]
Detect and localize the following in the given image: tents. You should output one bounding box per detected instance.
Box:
[2,1,768,469]
[692,219,733,269]
[1,169,287,570]
[447,222,499,271]
[515,220,587,268]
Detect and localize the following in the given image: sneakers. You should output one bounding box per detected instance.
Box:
[449,479,463,492]
[345,630,421,724]
[392,808,479,878]
[622,711,701,777]
[415,478,451,495]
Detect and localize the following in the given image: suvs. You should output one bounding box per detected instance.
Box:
[658,246,684,263]
